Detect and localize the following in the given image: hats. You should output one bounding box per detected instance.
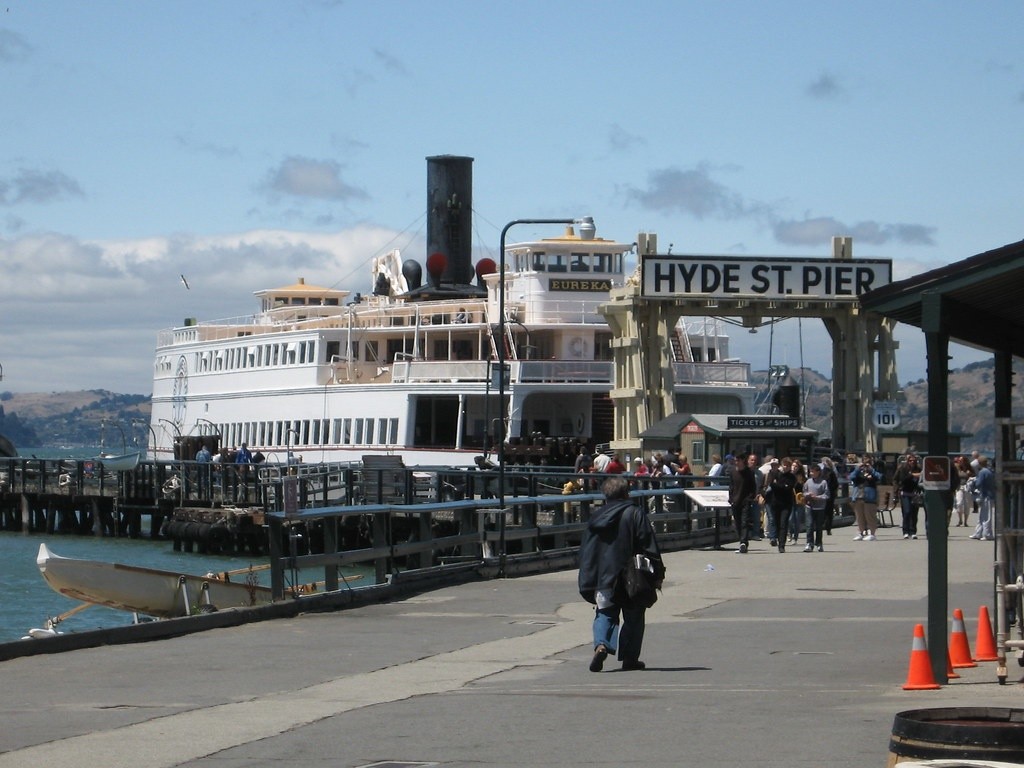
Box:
[634,457,642,462]
[769,458,778,465]
[725,454,734,460]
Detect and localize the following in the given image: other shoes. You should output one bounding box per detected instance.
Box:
[969,534,979,539]
[590,645,607,672]
[817,545,824,551]
[981,537,992,541]
[911,535,918,539]
[802,542,813,552]
[788,536,796,544]
[863,535,876,541]
[621,658,645,670]
[903,533,909,539]
[740,542,747,553]
[778,546,785,553]
[828,532,832,535]
[771,539,777,547]
[957,521,968,527]
[852,535,862,541]
[749,535,762,540]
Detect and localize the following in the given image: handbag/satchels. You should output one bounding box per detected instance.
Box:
[609,568,658,610]
[762,484,773,503]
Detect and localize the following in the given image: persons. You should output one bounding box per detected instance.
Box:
[578,475,667,672]
[195,443,265,501]
[574,443,995,554]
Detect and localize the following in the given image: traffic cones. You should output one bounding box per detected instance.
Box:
[970,606,999,663]
[949,609,977,668]
[947,649,962,678]
[902,624,941,689]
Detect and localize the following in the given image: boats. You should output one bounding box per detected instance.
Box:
[146,154,756,482]
[91,452,140,470]
[163,475,188,501]
[37,544,301,618]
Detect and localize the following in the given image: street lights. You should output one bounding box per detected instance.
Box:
[498,216,596,577]
[100,418,126,502]
[130,418,156,502]
[287,428,299,476]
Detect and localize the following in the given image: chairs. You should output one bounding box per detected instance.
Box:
[875,490,898,528]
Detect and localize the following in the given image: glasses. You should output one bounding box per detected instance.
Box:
[908,460,916,462]
